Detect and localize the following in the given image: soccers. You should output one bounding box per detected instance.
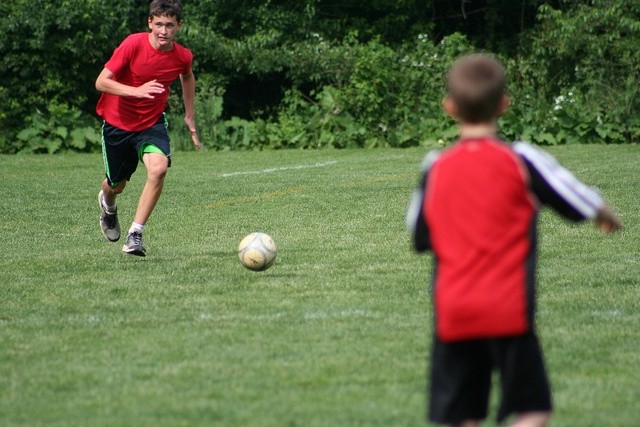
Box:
[238,232,277,272]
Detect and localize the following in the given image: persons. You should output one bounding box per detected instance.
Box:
[406,54,622,426]
[94,0,200,257]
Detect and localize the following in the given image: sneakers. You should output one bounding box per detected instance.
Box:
[122,232,147,257]
[99,190,121,242]
